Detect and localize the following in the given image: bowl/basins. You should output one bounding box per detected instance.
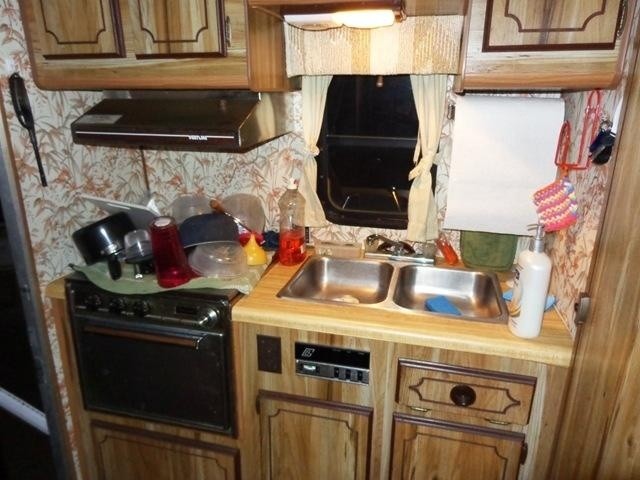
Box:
[187,243,249,280]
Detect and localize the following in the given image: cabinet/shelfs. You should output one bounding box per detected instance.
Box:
[231,320,573,480]
[17,0,300,92]
[453,0,637,95]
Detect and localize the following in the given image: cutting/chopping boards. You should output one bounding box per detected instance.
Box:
[80,194,159,225]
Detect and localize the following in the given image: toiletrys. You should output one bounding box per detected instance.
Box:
[508,223,554,339]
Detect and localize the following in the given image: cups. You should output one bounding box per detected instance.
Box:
[147,216,194,287]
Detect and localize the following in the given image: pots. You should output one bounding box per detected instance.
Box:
[71,213,139,280]
[125,213,242,264]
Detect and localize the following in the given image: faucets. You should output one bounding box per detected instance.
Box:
[367,233,416,256]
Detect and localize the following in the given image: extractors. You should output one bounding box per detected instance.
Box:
[71,90,296,153]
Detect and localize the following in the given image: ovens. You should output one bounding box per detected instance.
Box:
[63,277,239,439]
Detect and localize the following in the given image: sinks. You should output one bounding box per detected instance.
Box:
[393,264,508,325]
[276,254,394,310]
